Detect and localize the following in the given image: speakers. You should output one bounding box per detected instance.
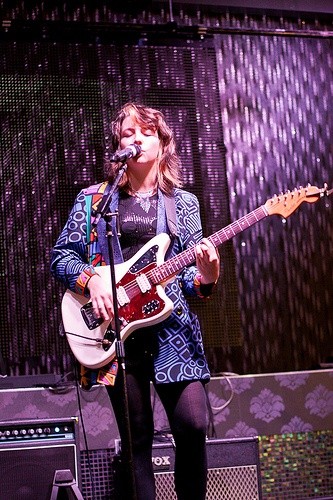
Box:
[0,417,82,500]
[152,435,263,500]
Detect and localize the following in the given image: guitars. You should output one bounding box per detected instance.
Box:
[61,182,333,370]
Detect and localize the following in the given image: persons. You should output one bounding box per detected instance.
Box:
[48,99,220,500]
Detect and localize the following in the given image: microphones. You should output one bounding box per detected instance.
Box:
[110,143,141,162]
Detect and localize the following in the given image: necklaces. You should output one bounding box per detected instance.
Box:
[128,185,158,195]
